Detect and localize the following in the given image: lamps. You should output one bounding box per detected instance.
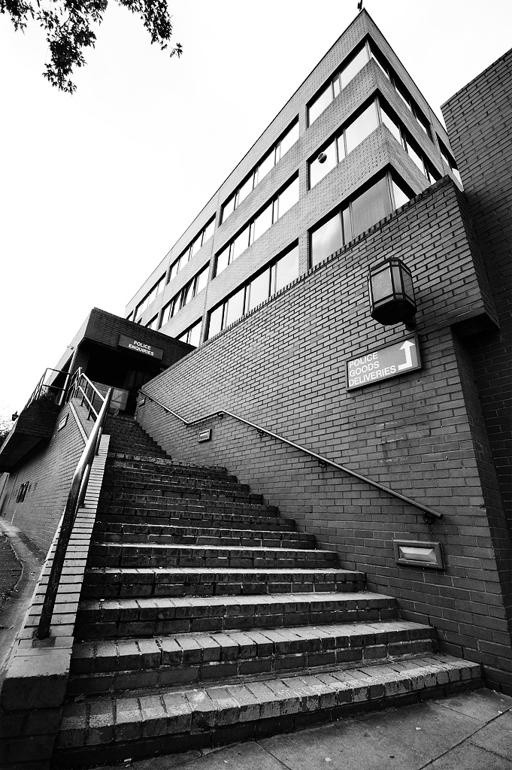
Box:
[363,254,417,333]
